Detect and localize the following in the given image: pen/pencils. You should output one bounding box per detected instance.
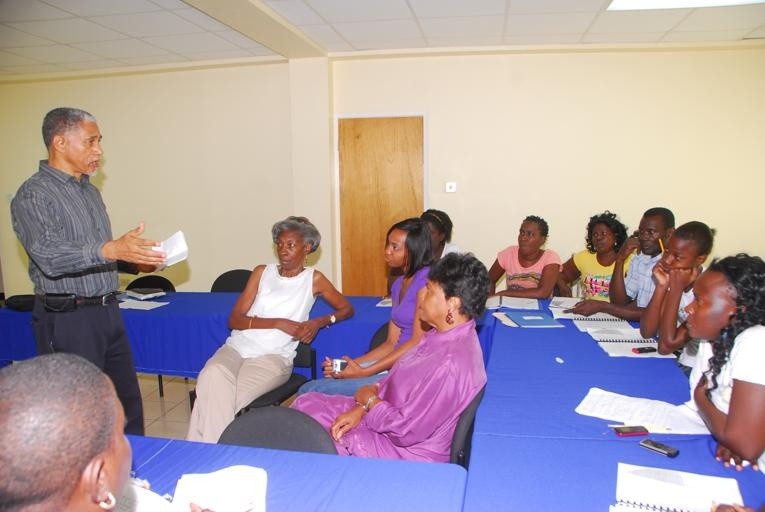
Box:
[563,307,574,314]
[658,238,665,253]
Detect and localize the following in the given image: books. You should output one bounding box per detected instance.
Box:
[570,303,634,333]
[152,230,188,271]
[127,286,166,300]
[548,297,584,320]
[589,328,675,359]
[485,295,540,311]
[609,463,744,512]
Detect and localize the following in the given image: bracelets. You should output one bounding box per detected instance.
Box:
[364,395,378,410]
[248,316,253,329]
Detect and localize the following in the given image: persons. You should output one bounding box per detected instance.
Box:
[488,215,561,298]
[557,213,627,301]
[297,217,440,396]
[186,217,355,445]
[418,210,465,258]
[639,221,714,354]
[290,253,489,462]
[564,208,675,321]
[685,254,765,476]
[0,352,132,512]
[10,108,167,437]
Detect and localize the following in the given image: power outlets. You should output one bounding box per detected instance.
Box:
[446,182,456,193]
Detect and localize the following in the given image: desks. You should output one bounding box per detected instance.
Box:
[0,292,499,379]
[123,434,468,512]
[462,299,765,512]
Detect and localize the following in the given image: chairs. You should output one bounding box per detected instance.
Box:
[449,385,485,467]
[211,269,252,291]
[216,406,339,455]
[365,323,390,353]
[125,275,187,397]
[189,342,316,416]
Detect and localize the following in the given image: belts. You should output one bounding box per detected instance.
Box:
[38,291,116,306]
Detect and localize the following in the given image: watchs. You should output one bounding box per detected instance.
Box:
[328,312,337,325]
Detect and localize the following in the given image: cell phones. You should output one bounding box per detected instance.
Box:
[332,357,347,372]
[640,439,681,459]
[631,346,656,353]
[611,425,650,437]
